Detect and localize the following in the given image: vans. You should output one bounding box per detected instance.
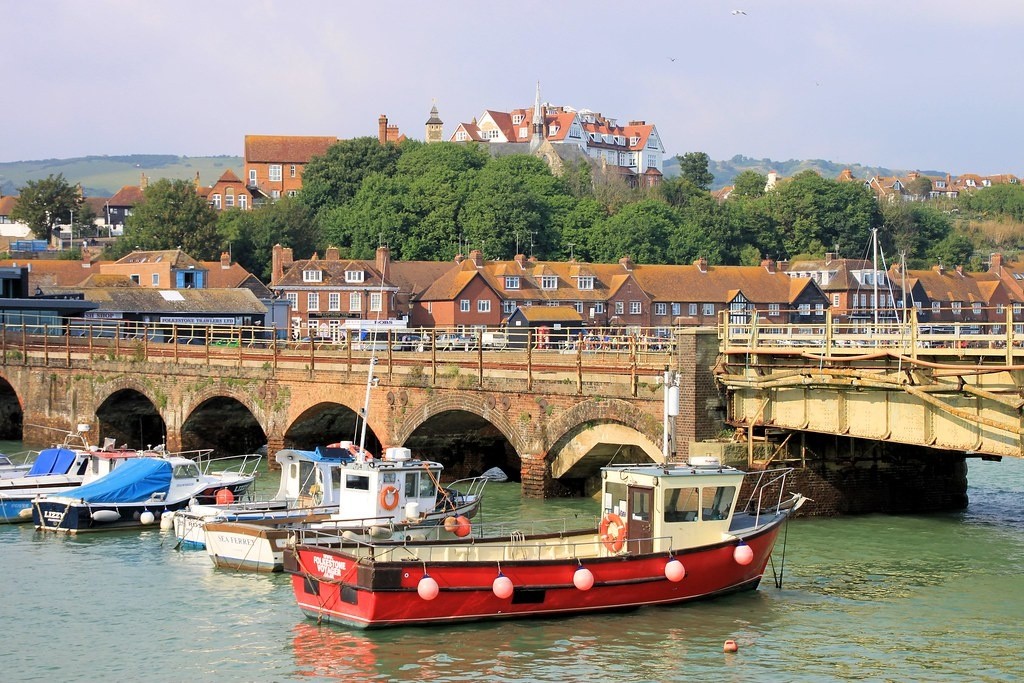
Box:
[482,332,510,350]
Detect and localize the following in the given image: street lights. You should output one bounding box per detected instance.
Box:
[69,210,74,251]
[104,199,112,238]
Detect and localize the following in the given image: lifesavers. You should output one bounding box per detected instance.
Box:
[382,487,399,509]
[600,514,625,551]
[349,445,373,461]
[327,442,341,448]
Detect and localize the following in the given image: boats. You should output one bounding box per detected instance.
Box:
[29,454,263,533]
[0,422,168,524]
[203,447,490,572]
[290,373,815,631]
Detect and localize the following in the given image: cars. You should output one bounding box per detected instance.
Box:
[468,337,480,347]
[209,338,239,347]
[302,337,333,342]
[173,337,206,344]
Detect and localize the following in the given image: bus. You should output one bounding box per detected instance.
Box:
[350,329,438,351]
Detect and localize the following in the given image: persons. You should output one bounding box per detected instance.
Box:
[99,227,102,237]
[83,240,89,253]
[570,331,676,351]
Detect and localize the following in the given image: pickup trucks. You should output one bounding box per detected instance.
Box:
[436,333,469,349]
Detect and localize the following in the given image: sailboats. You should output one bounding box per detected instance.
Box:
[171,258,382,548]
